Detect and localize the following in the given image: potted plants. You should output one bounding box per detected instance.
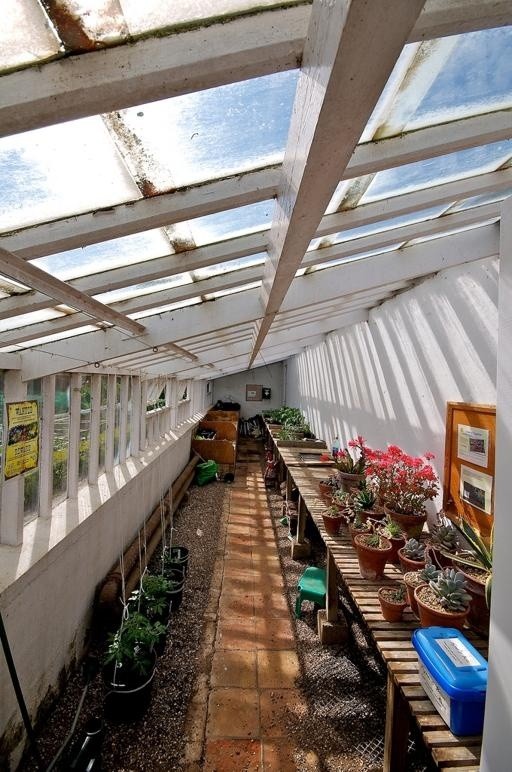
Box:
[265,406,316,440]
[322,511,495,629]
[319,435,440,513]
[102,542,193,718]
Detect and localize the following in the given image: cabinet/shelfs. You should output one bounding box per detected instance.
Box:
[189,400,240,479]
